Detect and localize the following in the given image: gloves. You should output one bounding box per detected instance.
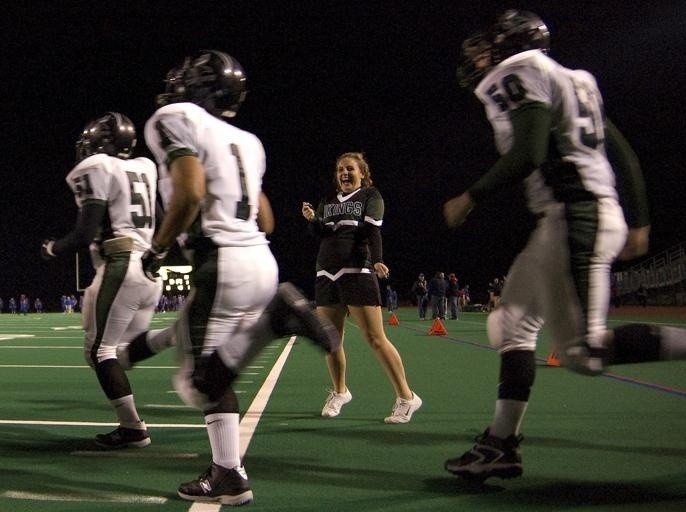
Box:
[40,239,58,261]
[141,237,171,281]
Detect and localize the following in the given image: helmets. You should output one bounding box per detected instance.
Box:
[73,112,138,158]
[157,48,246,119]
[451,9,551,89]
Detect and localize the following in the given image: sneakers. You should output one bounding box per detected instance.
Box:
[177,461,254,506]
[444,428,525,480]
[94,417,151,449]
[384,391,423,423]
[264,281,342,355]
[322,387,352,417]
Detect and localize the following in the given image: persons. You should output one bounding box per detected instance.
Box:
[1,288,78,315]
[441,7,686,479]
[141,48,339,503]
[39,111,178,447]
[157,294,186,313]
[386,268,507,320]
[300,153,423,424]
